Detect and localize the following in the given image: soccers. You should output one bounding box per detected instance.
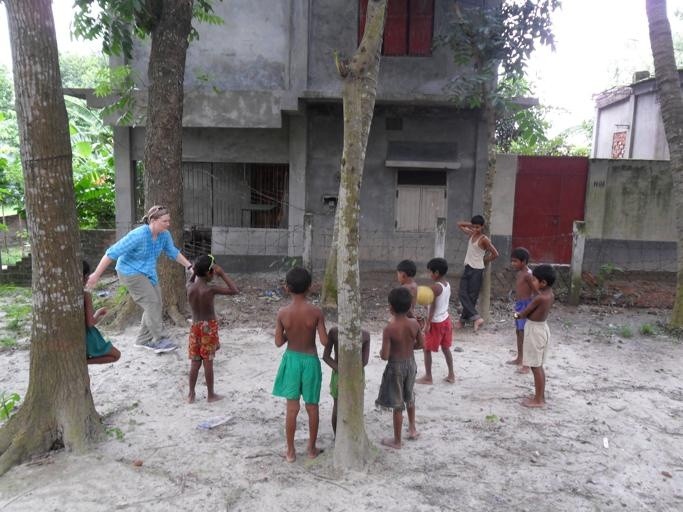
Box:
[416,287,434,306]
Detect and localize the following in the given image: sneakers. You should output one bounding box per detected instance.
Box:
[154,339,178,354]
[134,341,156,350]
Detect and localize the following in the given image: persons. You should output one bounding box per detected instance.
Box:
[375,287,421,449]
[270,265,328,462]
[512,264,556,408]
[415,258,456,385]
[506,246,541,375]
[394,259,419,318]
[81,260,121,365]
[321,289,370,442]
[454,213,500,334]
[185,253,239,404]
[85,204,195,355]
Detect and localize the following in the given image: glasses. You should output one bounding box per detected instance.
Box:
[158,205,168,211]
